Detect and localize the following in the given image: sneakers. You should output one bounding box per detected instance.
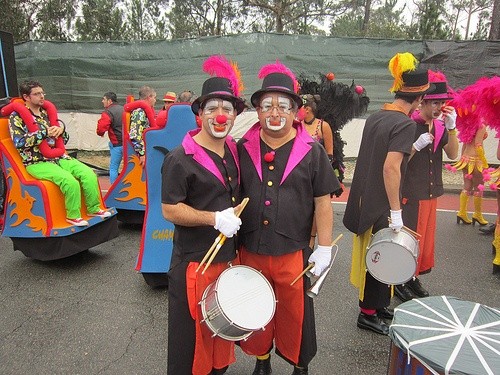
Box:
[65,218,88,226]
[88,210,111,216]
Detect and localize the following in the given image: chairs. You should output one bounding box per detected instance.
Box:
[0,97,118,238]
[101,96,161,211]
[136,103,200,273]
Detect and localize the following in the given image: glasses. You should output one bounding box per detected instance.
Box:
[30,91,47,97]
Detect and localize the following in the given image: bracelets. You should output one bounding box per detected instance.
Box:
[447,130,456,135]
[310,234,317,237]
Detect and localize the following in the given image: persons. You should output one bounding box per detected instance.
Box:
[235,72,343,375]
[456,122,489,226]
[7,79,112,227]
[96,71,335,252]
[394,81,458,303]
[160,76,241,375]
[341,68,436,336]
[491,138,500,273]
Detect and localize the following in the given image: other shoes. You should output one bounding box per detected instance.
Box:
[480,223,496,233]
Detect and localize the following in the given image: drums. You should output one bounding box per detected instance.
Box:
[365,228,420,286]
[201,265,276,341]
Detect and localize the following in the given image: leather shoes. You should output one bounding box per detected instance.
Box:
[375,305,395,319]
[253,354,273,375]
[358,311,390,335]
[393,284,415,301]
[292,366,309,375]
[406,277,429,297]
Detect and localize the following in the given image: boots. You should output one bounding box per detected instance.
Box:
[472,195,488,225]
[457,191,473,224]
[493,224,500,273]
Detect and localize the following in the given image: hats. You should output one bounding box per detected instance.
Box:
[251,61,303,109]
[163,91,177,101]
[191,56,245,117]
[422,68,453,100]
[389,52,430,95]
